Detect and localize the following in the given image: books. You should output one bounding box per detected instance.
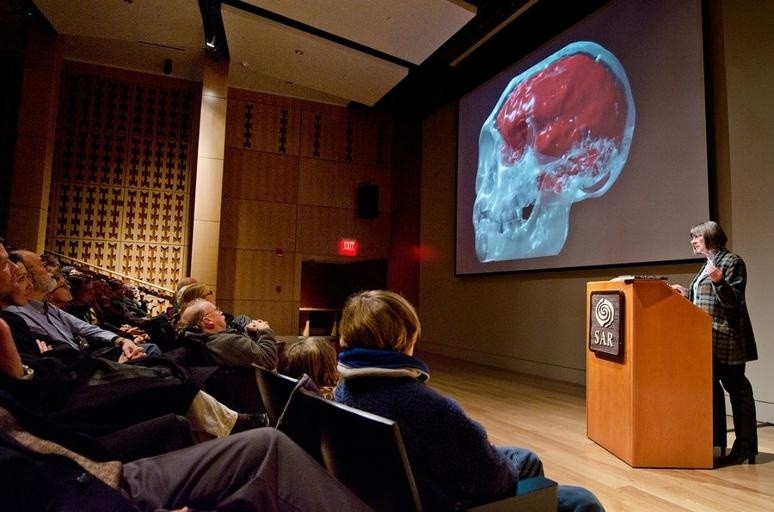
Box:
[618,276,668,280]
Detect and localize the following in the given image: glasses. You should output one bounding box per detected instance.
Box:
[202,290,213,298]
[26,262,47,272]
[205,305,222,316]
[60,280,71,287]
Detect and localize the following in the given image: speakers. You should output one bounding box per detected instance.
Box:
[357,184,379,219]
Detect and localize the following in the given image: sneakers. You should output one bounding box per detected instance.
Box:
[230,412,269,435]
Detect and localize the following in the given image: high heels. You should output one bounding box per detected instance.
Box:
[712,439,759,464]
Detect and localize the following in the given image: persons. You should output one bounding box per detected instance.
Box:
[670,220,759,467]
[1,239,368,512]
[335,291,603,511]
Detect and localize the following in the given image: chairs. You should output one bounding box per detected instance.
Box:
[251,361,558,512]
